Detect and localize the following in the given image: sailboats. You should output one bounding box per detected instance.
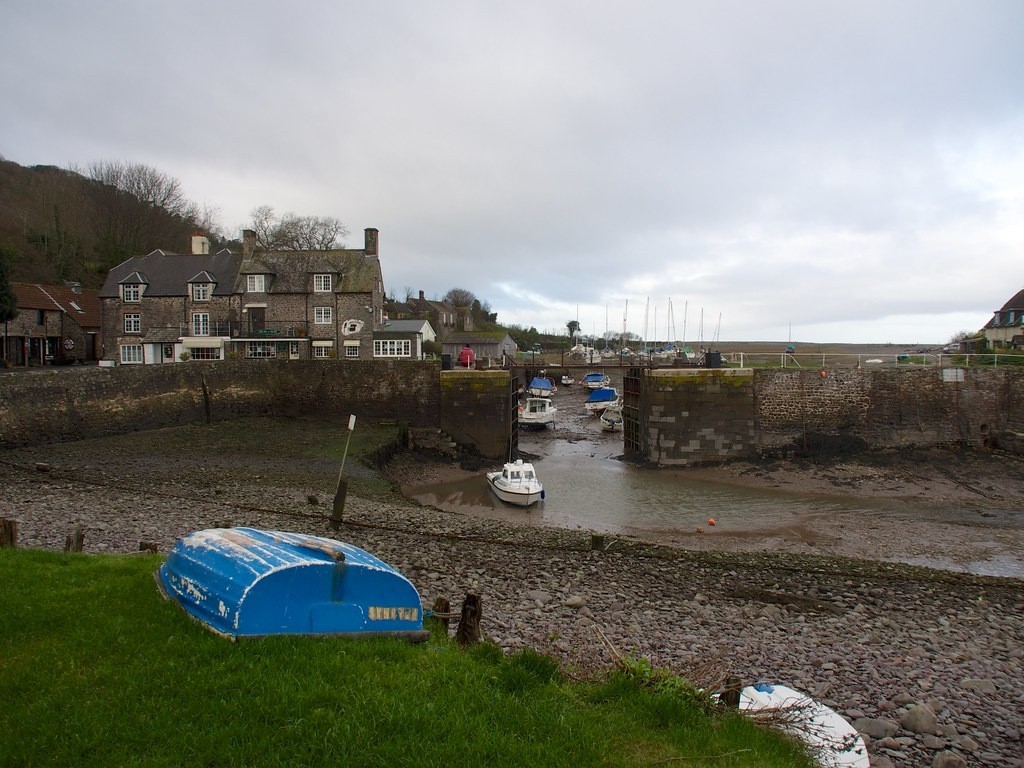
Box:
[570,295,731,366]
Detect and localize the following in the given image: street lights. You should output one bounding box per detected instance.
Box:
[532,347,535,370]
[560,345,564,367]
[589,348,594,368]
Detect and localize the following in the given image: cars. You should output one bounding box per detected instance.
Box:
[942,343,961,354]
[904,347,943,353]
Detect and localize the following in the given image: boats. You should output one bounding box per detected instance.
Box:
[561,369,577,385]
[526,377,558,399]
[485,458,546,511]
[518,398,557,431]
[579,369,611,390]
[583,388,620,417]
[600,404,624,432]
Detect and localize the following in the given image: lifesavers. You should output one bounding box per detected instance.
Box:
[64,339,74,350]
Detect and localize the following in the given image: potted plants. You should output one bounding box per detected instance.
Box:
[297,326,305,337]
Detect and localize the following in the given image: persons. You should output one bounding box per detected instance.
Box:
[787,348,795,363]
